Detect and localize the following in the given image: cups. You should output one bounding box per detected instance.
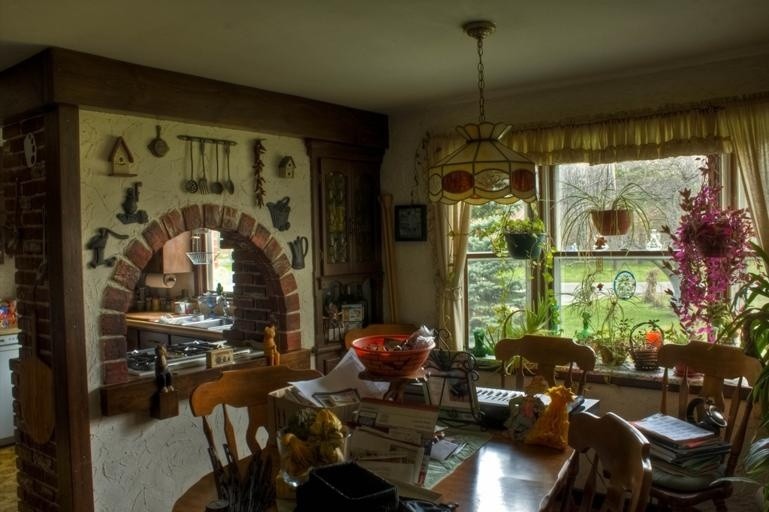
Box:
[174,302,185,315]
[185,302,192,314]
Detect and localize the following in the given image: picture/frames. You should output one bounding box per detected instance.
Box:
[394,204,427,242]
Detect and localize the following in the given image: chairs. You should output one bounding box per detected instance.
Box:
[636,340,764,511]
[341,321,418,351]
[192,364,321,463]
[568,410,652,511]
[493,336,595,397]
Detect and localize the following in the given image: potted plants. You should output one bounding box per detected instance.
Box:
[591,284,634,366]
[545,170,662,279]
[628,318,666,370]
[477,297,559,373]
[443,204,559,305]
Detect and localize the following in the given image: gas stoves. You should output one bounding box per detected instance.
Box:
[128,339,235,371]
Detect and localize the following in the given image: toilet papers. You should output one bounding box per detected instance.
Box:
[144,272,176,290]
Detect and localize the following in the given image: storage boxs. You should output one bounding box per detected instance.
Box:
[266,379,360,437]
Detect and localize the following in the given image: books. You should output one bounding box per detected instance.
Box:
[627,411,735,477]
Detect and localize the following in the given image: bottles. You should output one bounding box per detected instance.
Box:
[399,325,431,351]
[199,293,208,316]
[324,285,369,343]
[135,288,196,312]
[646,229,663,251]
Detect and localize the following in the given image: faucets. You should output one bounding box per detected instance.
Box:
[215,295,230,317]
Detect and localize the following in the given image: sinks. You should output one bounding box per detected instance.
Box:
[154,312,220,326]
[184,315,235,332]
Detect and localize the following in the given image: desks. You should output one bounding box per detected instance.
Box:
[172,437,578,511]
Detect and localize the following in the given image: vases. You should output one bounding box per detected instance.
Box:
[692,230,731,258]
[673,358,701,378]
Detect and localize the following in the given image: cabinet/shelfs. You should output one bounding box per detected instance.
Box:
[313,274,384,351]
[126,328,191,351]
[142,230,192,273]
[306,141,381,274]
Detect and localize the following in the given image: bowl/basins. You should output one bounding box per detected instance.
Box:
[352,335,435,375]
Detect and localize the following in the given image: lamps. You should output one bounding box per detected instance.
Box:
[425,16,538,204]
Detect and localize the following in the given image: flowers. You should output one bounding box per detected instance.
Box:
[662,157,757,341]
